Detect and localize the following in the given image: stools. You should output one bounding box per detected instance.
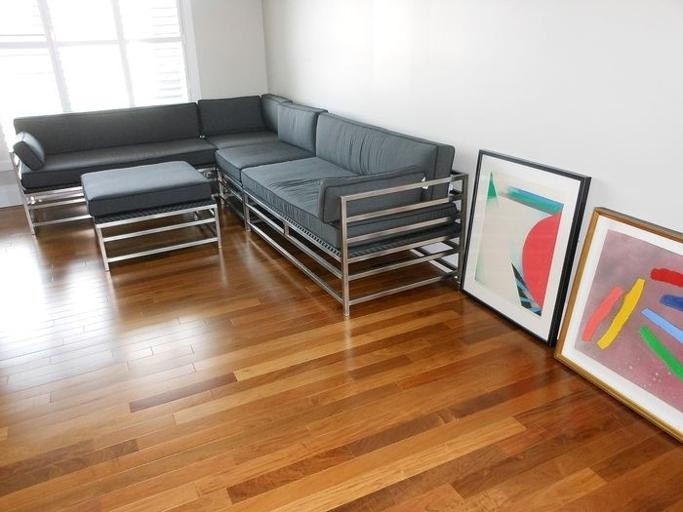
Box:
[78,160,222,270]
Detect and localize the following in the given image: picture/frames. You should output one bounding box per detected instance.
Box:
[551,208,682,445]
[461,145,593,348]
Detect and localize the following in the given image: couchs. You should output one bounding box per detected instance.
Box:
[7,93,468,319]
